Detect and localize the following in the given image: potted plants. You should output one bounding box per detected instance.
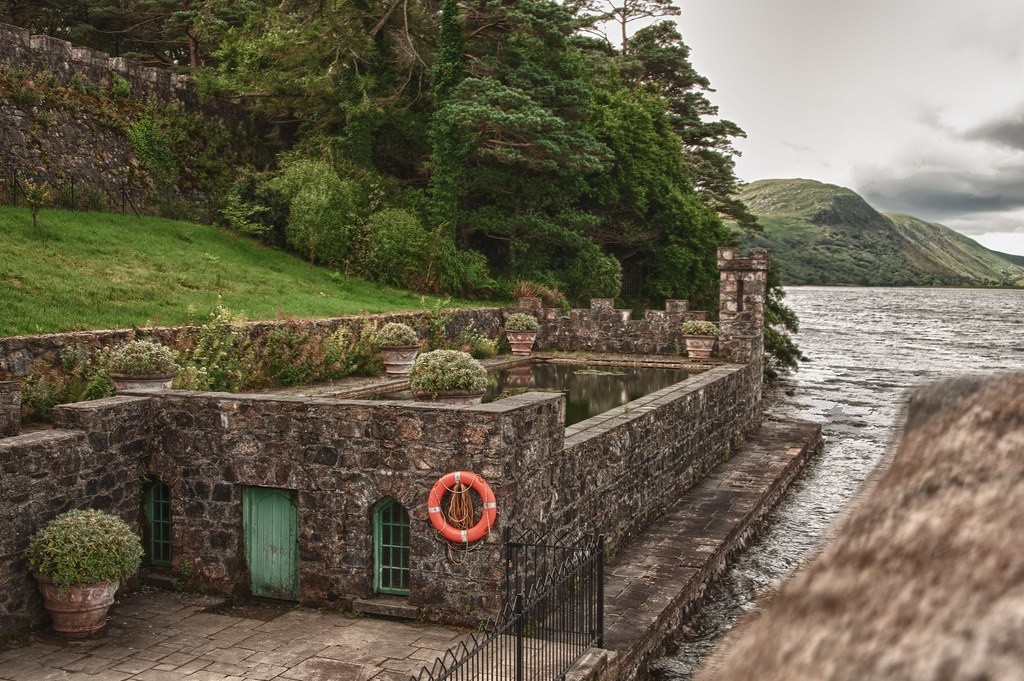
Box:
[21,507,146,639]
[375,323,421,377]
[505,365,536,388]
[681,320,720,360]
[504,313,540,356]
[409,350,488,405]
[107,339,179,390]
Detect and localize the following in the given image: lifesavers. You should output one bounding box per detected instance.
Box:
[428,470,497,542]
